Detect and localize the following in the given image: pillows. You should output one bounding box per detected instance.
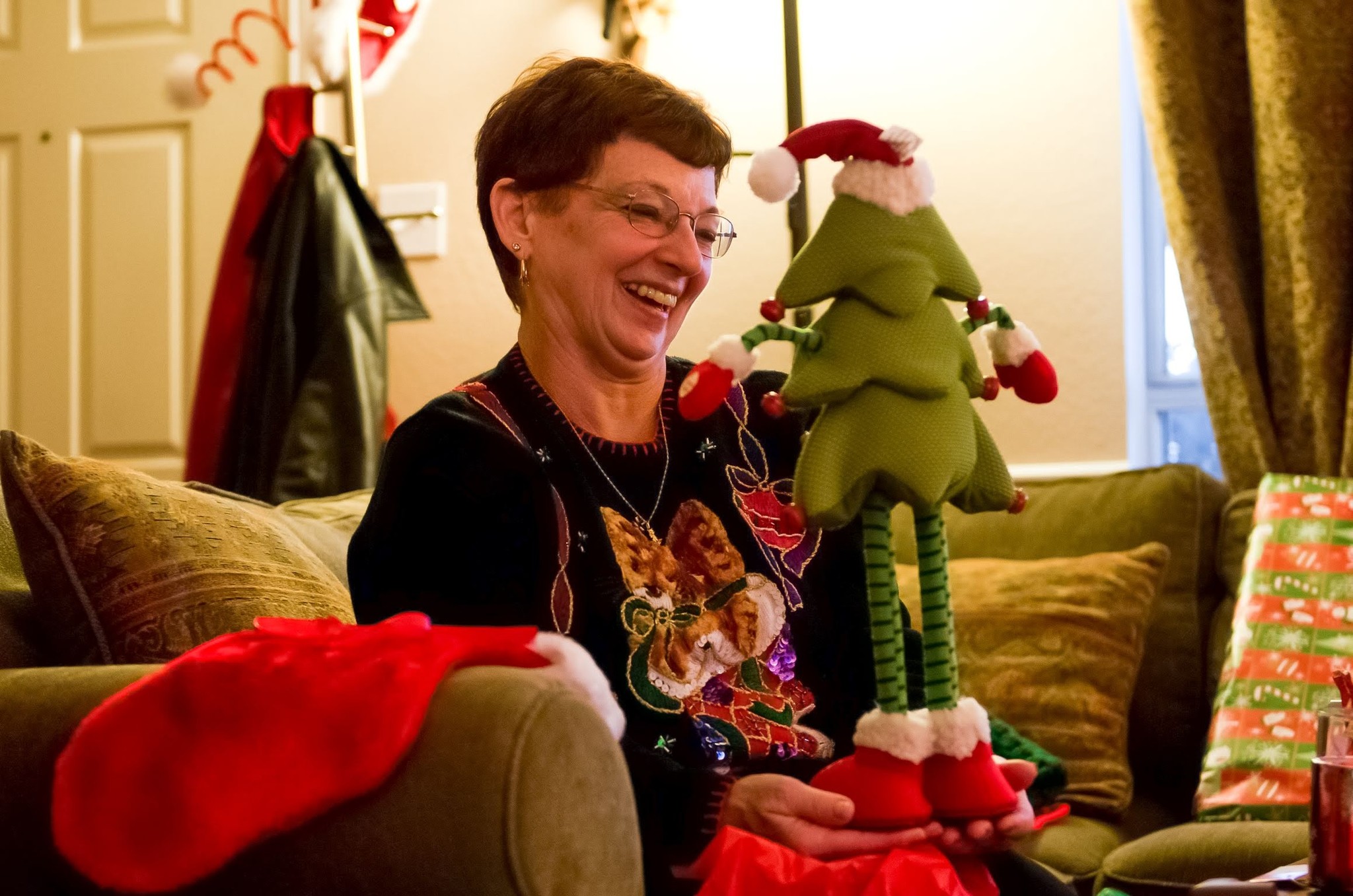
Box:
[892,540,1171,814]
[0,422,352,664]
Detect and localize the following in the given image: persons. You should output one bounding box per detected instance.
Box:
[346,57,1035,896]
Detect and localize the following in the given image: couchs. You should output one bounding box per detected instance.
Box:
[0,461,1310,896]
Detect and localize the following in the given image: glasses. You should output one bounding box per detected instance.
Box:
[566,182,737,258]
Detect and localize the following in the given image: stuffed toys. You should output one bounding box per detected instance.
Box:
[675,118,1061,828]
